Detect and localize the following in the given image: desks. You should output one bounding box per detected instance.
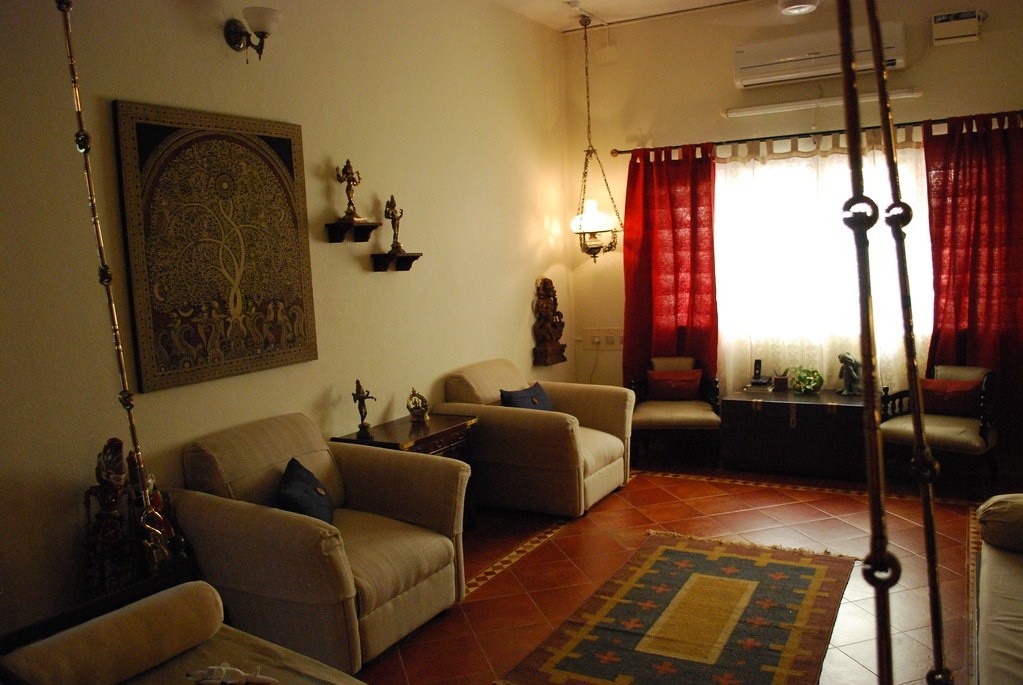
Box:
[329,414,480,527]
[720,388,868,482]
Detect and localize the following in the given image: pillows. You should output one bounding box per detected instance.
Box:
[647,369,702,401]
[918,376,981,417]
[500,382,554,411]
[276,457,335,524]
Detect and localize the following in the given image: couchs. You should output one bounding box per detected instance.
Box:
[159,410,471,676]
[430,358,636,518]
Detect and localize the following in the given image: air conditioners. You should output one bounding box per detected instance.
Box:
[733,21,907,88]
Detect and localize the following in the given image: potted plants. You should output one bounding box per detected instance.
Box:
[789,366,825,395]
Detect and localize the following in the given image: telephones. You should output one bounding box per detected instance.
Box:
[751,358,772,385]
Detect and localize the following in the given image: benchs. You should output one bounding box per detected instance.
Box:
[0,573,367,685]
[973,493,1023,685]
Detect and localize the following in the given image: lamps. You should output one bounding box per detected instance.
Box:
[570,14,624,263]
[721,85,924,119]
[223,6,278,63]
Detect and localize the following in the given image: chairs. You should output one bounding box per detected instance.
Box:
[626,356,721,470]
[880,364,1003,502]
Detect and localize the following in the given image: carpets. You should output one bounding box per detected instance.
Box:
[494,529,863,685]
